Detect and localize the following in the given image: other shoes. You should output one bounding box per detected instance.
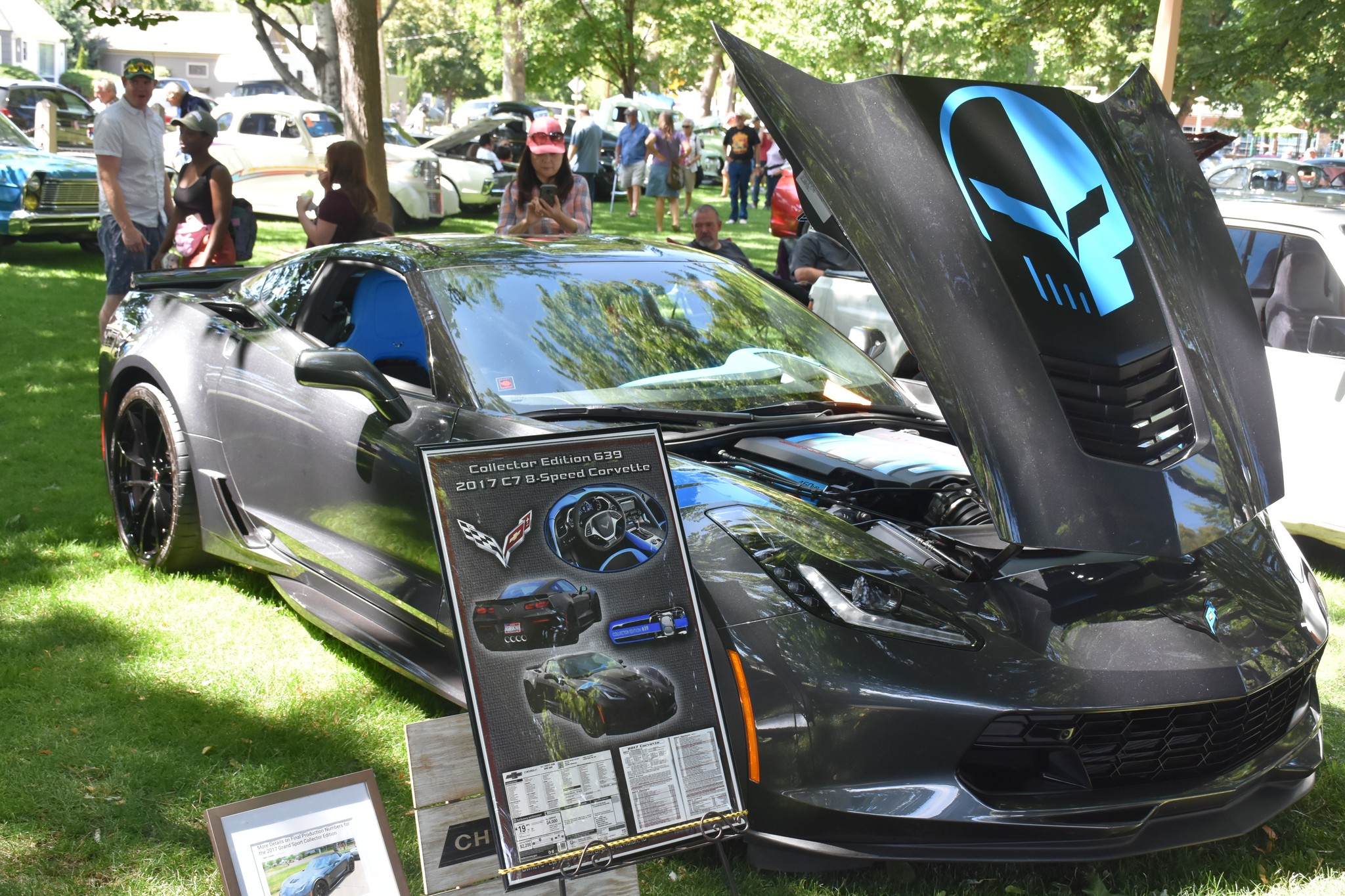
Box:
[763,205,771,211]
[752,200,759,210]
[682,209,688,218]
[667,211,672,215]
[627,211,637,217]
[725,219,736,224]
[738,218,748,224]
[672,224,680,232]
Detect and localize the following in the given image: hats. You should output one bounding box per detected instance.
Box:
[733,108,752,120]
[721,112,736,124]
[124,58,156,81]
[1307,147,1316,153]
[527,115,566,154]
[170,110,219,138]
[623,107,639,116]
[752,116,760,123]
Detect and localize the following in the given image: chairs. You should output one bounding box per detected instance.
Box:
[335,269,429,373]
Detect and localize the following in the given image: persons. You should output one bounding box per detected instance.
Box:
[789,223,864,311]
[93,58,174,345]
[1281,146,1294,160]
[476,133,512,172]
[614,106,651,217]
[163,80,211,119]
[296,140,379,249]
[666,118,703,217]
[681,204,755,323]
[645,111,692,232]
[716,107,785,224]
[93,78,120,106]
[567,104,603,225]
[150,110,235,269]
[495,116,593,235]
[1303,148,1317,159]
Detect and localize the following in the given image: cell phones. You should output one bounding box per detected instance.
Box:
[539,184,557,211]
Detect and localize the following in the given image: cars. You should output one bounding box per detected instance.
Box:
[808,199,1345,550]
[1202,157,1345,208]
[161,94,443,225]
[150,76,198,128]
[0,77,103,144]
[1196,152,1236,187]
[404,91,747,212]
[0,110,113,255]
[230,79,291,96]
[1287,158,1344,192]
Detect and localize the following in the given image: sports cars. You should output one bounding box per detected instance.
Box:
[98,27,1326,873]
[524,650,678,741]
[470,575,603,652]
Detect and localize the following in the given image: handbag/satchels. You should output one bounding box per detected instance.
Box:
[694,165,705,188]
[666,160,684,188]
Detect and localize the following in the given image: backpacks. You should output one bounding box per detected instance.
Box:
[181,162,258,262]
[327,189,395,243]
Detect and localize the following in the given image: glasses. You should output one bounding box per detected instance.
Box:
[526,131,565,146]
[682,125,690,128]
